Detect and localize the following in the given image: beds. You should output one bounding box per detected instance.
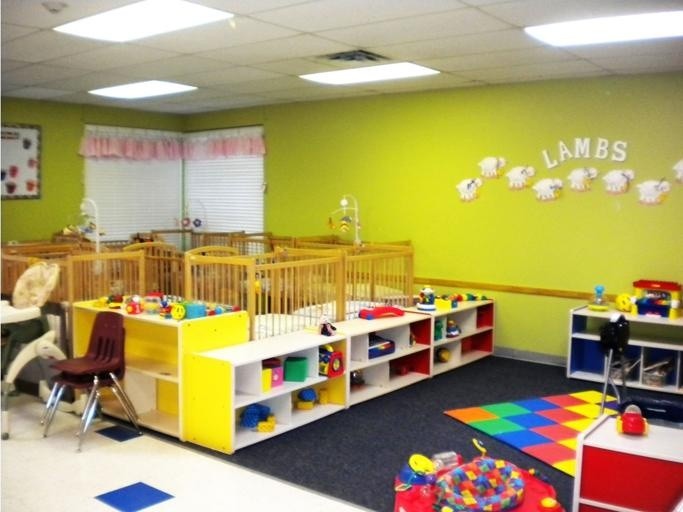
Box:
[183,232,414,338]
[2,231,183,304]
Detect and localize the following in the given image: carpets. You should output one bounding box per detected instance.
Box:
[107,354,683,512]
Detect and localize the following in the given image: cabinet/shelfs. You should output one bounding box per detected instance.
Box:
[349,316,433,409]
[74,299,180,442]
[184,338,348,454]
[433,299,493,378]
[573,414,683,511]
[566,303,682,395]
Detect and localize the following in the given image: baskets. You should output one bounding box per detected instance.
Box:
[609,364,631,379]
[642,359,670,388]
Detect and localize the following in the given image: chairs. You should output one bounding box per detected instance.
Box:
[1,262,141,443]
